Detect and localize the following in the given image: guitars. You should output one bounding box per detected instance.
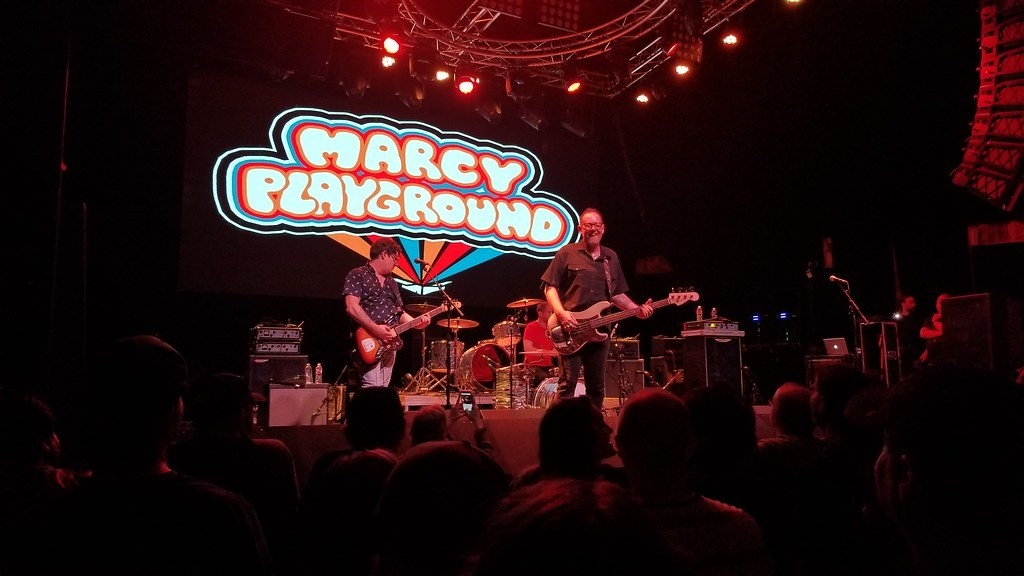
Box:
[547,286,700,356]
[355,298,462,366]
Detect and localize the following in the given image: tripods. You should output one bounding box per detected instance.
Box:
[402,325,479,398]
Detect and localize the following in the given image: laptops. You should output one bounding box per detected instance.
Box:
[823,338,851,355]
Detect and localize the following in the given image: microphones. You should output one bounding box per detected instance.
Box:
[415,258,429,265]
[830,275,848,284]
[481,356,501,368]
[636,370,645,374]
[312,413,320,416]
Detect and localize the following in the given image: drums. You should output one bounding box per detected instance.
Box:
[532,375,588,410]
[458,342,513,393]
[428,340,464,374]
[491,321,521,347]
[549,364,586,380]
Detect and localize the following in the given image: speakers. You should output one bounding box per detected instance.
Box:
[940,295,1024,382]
[603,359,645,398]
[683,335,743,397]
[269,384,328,426]
[968,0,1024,211]
[249,354,309,428]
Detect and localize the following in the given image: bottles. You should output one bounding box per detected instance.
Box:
[305,363,313,384]
[711,307,717,319]
[697,306,702,321]
[315,363,322,384]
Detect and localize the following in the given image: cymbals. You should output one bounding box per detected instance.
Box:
[507,298,543,308]
[405,303,436,314]
[436,318,482,329]
[518,349,559,357]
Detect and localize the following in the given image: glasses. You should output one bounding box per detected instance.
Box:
[580,223,603,230]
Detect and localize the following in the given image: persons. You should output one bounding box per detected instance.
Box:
[541,207,653,410]
[0,336,1023,576]
[521,302,554,389]
[341,242,432,390]
[879,292,953,370]
